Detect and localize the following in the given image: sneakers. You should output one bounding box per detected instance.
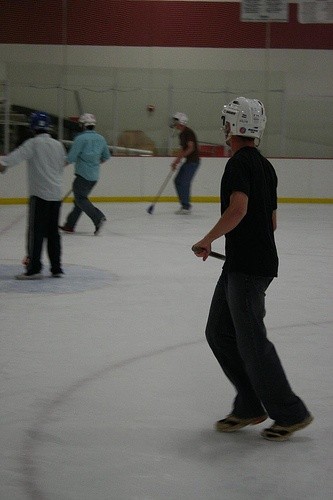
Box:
[215,411,268,433]
[261,414,314,441]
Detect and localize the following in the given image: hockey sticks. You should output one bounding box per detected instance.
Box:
[146,167,176,216]
[62,187,72,203]
[190,245,226,261]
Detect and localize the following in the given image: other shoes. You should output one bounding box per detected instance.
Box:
[58,225,75,233]
[175,208,192,215]
[53,269,68,278]
[94,214,108,235]
[15,272,43,280]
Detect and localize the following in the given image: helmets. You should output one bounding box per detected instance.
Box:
[30,112,53,134]
[219,97,267,146]
[173,112,189,127]
[77,113,96,130]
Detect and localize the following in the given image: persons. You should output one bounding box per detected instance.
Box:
[0,113,65,281]
[58,113,110,235]
[170,112,200,215]
[192,96,313,441]
